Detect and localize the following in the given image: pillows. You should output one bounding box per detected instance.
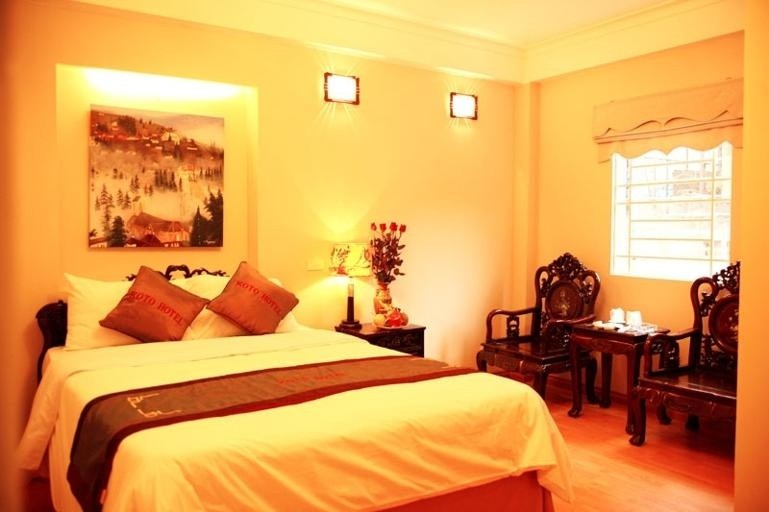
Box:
[59,271,198,350]
[104,266,210,340]
[190,272,300,334]
[207,261,300,336]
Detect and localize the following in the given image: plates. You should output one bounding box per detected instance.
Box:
[608,320,626,325]
[376,325,403,330]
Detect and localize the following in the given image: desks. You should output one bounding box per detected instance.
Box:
[564,321,671,434]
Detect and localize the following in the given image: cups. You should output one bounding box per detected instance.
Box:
[610,307,624,322]
[627,311,643,328]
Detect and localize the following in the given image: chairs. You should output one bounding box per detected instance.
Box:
[626,258,740,447]
[473,251,600,406]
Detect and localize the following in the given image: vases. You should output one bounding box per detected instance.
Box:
[374,281,391,313]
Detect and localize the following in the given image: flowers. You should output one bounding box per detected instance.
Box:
[365,222,408,283]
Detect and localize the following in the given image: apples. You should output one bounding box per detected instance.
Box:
[373,313,385,326]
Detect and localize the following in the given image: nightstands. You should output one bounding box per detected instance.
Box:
[334,319,426,358]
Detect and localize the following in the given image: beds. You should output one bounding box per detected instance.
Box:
[21,256,582,511]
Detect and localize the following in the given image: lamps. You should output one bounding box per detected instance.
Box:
[449,91,479,121]
[322,72,361,105]
[328,242,371,330]
